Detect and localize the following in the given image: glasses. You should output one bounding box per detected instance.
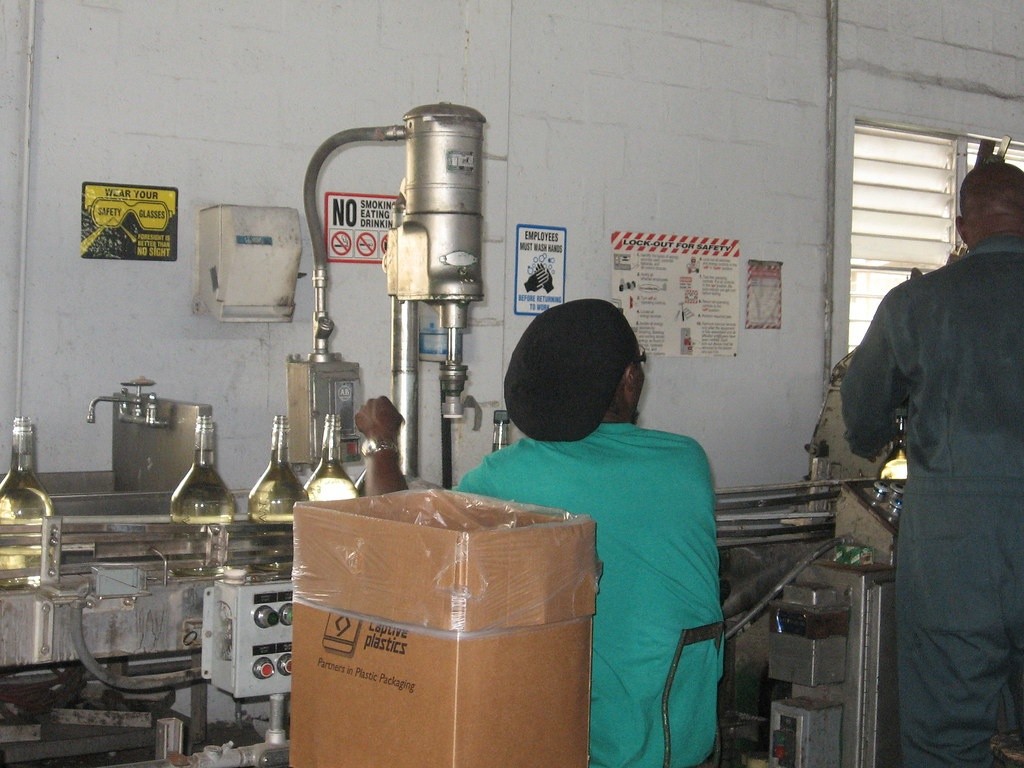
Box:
[630,344,646,367]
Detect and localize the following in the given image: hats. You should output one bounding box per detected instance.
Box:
[503,299,638,442]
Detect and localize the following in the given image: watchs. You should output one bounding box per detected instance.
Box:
[361,437,398,457]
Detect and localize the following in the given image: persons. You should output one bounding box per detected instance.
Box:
[354,298,723,768]
[840,162,1024,768]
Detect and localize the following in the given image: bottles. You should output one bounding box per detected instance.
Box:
[303,414,359,503]
[492,409,510,453]
[247,415,310,582]
[354,467,366,498]
[168,413,233,579]
[0,417,56,591]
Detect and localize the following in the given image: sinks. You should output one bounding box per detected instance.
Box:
[1,470,172,516]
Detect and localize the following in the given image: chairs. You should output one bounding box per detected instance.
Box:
[661,621,726,768]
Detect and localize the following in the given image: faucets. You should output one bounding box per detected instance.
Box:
[88,375,170,427]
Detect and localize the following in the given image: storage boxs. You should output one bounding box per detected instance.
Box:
[290,487,601,768]
